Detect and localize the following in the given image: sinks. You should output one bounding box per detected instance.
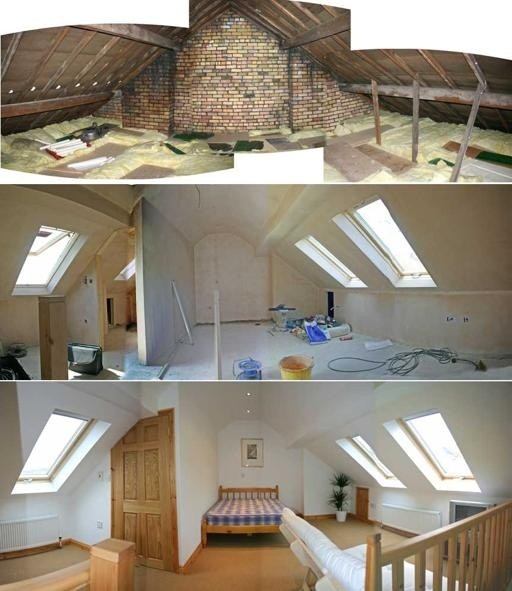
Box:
[0,539,91,590]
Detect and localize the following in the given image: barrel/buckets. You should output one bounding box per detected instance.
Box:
[278,354,315,379]
[238,360,263,380]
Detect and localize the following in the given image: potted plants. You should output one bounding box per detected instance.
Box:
[328,472,356,522]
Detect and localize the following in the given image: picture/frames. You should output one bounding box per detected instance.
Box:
[240,438,265,468]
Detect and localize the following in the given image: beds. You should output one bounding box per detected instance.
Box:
[202,484,304,546]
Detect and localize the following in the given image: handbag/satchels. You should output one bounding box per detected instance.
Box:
[67,343,102,375]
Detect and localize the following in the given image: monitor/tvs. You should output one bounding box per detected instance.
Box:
[448,500,496,545]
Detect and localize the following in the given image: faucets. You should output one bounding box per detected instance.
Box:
[55,534,65,548]
